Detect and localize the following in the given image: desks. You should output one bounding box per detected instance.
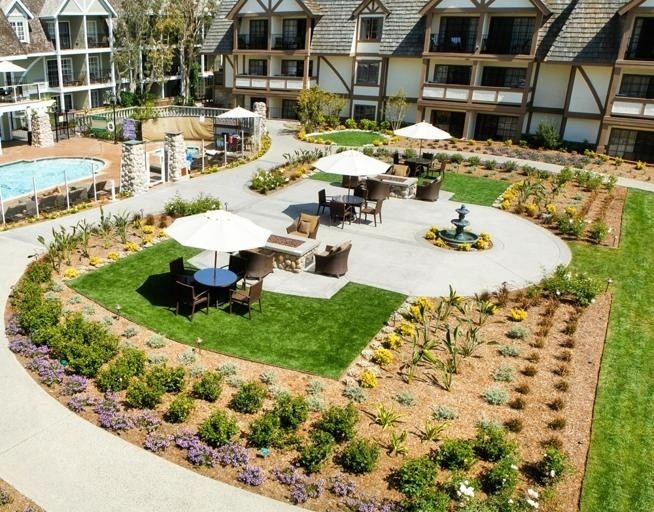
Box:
[261,232,321,274]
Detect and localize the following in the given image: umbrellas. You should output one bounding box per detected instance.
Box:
[165,209,274,279]
[218,106,263,156]
[0,61,27,73]
[393,119,453,157]
[312,148,391,196]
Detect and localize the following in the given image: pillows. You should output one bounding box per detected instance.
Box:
[298,221,312,235]
[298,212,320,233]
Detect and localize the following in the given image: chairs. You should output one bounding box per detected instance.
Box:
[169,248,276,323]
[286,215,321,239]
[314,240,353,279]
[316,152,447,229]
